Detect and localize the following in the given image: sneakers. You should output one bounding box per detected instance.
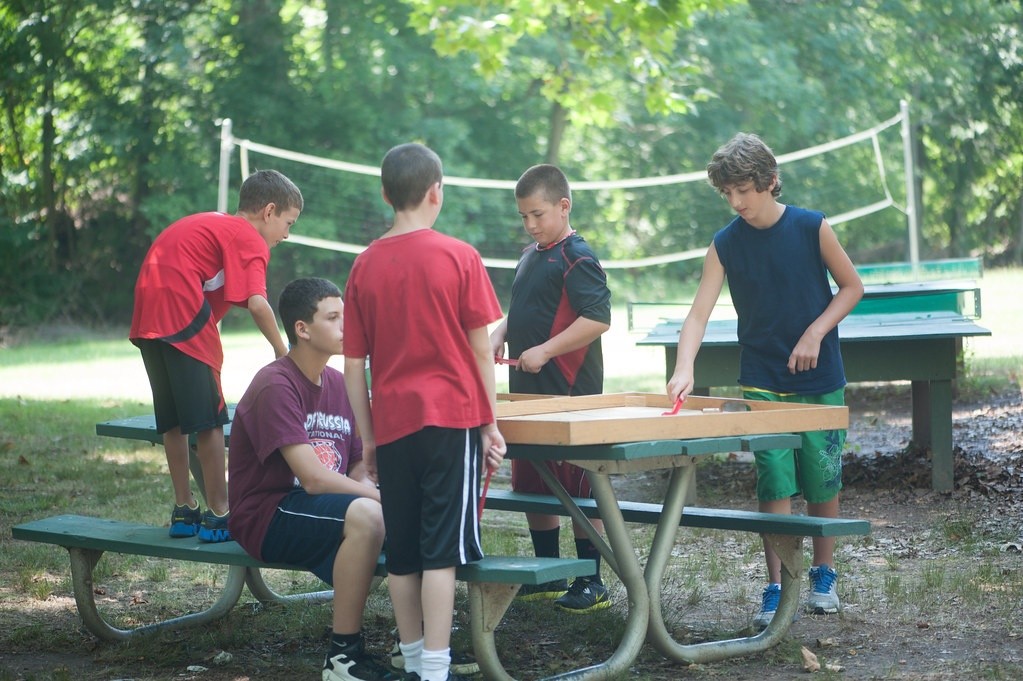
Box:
[401,668,421,681]
[447,671,472,681]
[807,570,840,613]
[198,509,234,543]
[555,578,612,613]
[168,499,203,537]
[322,638,400,681]
[755,588,797,626]
[391,639,482,674]
[515,578,568,601]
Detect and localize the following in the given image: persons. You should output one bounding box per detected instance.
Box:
[665,132,865,627]
[229,280,481,681]
[128,170,304,543]
[490,163,614,615]
[342,144,506,680]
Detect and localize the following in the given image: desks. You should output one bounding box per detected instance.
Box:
[106,392,805,658]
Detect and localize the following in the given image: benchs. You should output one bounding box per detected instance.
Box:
[20,510,601,677]
[478,483,871,653]
